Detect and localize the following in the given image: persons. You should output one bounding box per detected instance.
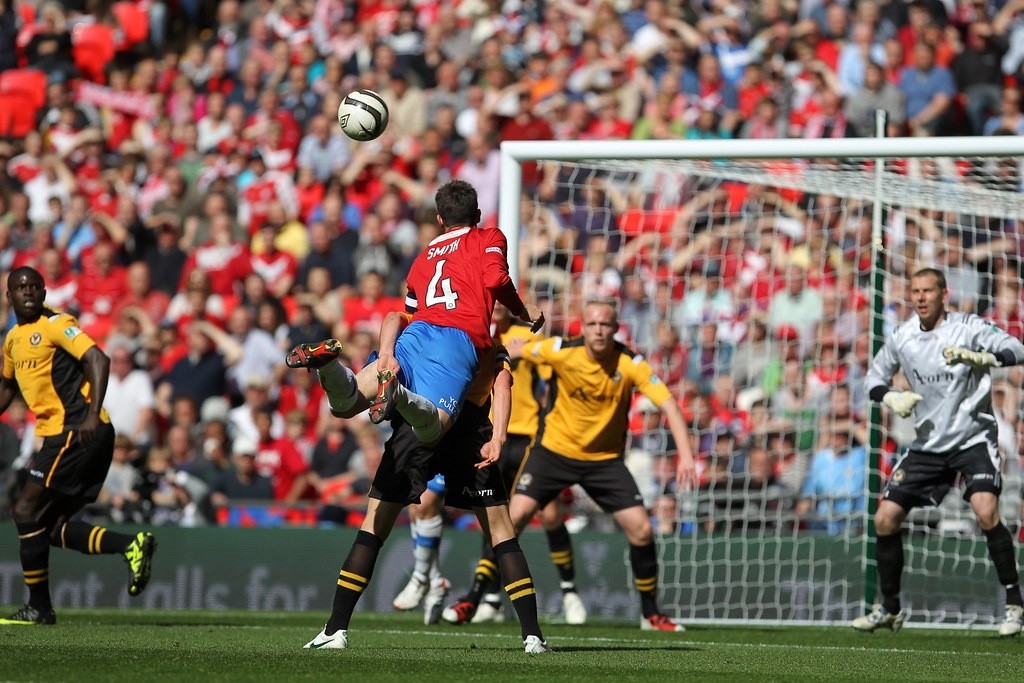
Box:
[0,0,1024,626]
[285,181,546,441]
[850,268,1024,636]
[304,312,551,654]
[439,303,697,631]
[0,265,158,625]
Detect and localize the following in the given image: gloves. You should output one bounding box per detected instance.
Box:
[941,345,1002,368]
[882,389,923,418]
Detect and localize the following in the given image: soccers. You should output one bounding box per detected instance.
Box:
[338,88,391,143]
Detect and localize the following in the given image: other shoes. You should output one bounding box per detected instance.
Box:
[369,367,401,425]
[469,598,507,624]
[562,592,587,625]
[285,338,345,370]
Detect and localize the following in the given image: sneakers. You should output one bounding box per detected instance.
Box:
[523,635,555,654]
[998,603,1024,636]
[442,595,474,622]
[850,600,907,634]
[640,614,687,633]
[391,568,441,610]
[0,603,56,627]
[123,529,159,597]
[423,578,453,625]
[301,622,348,650]
